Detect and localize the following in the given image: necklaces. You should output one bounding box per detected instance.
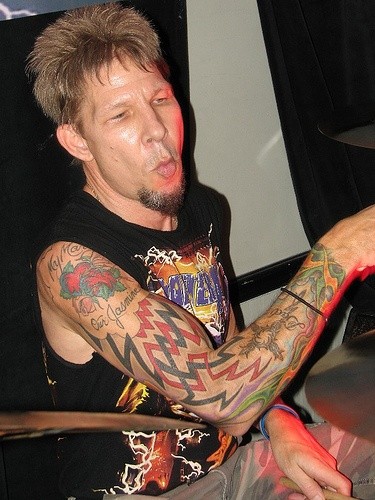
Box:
[86,183,101,202]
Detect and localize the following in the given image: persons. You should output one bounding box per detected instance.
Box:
[23,3,375,500]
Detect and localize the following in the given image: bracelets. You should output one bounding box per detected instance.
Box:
[281,288,328,323]
[259,404,300,442]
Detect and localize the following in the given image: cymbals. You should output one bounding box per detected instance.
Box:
[304,330,375,445]
[0,409,208,441]
[317,101,375,150]
